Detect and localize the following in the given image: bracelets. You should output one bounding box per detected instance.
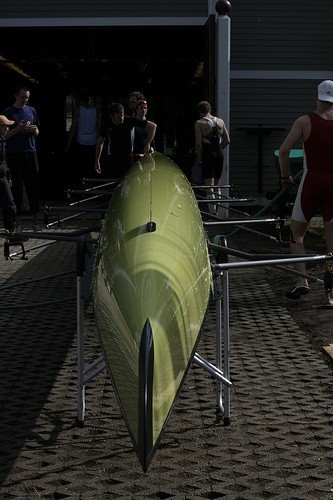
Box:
[280,175,290,180]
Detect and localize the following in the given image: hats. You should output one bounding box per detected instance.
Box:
[135,100,147,110]
[317,80,333,103]
[0,115,15,125]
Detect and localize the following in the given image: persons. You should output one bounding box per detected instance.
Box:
[0,115,30,244]
[59,91,157,204]
[194,101,231,222]
[4,84,41,216]
[279,79,333,308]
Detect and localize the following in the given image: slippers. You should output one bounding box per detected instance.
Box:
[286,285,311,300]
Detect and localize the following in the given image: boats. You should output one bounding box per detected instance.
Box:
[90,151,213,472]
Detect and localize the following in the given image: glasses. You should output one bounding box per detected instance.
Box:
[128,99,138,103]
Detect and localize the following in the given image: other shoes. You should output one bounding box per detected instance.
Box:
[207,216,217,222]
[9,238,30,244]
[33,220,42,233]
